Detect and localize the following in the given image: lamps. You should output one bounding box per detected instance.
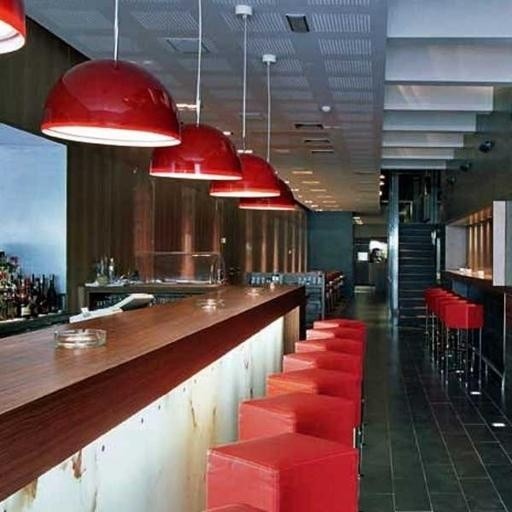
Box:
[237,53,295,210]
[0,0,27,54]
[208,4,281,198]
[149,0,244,181]
[38,0,182,147]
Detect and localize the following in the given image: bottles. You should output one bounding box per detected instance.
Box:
[247,270,323,286]
[98,253,117,284]
[0,249,61,319]
[306,287,321,301]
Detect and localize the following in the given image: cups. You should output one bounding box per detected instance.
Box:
[459,268,485,279]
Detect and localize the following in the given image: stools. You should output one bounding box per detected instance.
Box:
[423,287,484,393]
[205,318,368,512]
